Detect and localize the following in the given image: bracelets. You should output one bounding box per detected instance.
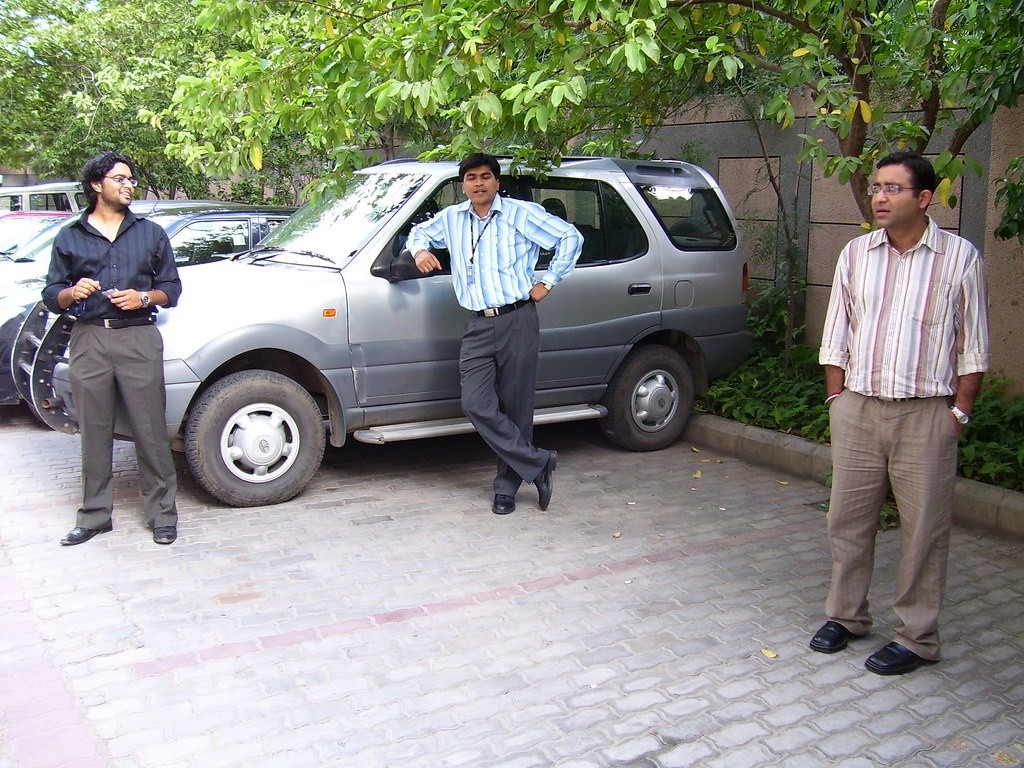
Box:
[825,394,839,403]
[540,281,553,292]
[72,286,80,304]
[415,250,427,258]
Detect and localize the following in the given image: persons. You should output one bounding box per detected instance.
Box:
[40,152,182,546]
[809,149,989,676]
[406,152,584,516]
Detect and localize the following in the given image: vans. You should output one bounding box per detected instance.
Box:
[0,182,89,215]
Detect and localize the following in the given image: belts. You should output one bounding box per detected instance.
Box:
[477,297,532,317]
[876,396,936,403]
[77,315,156,329]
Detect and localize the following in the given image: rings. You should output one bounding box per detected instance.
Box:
[121,303,124,307]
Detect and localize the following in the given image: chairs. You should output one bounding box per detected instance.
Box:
[541,198,569,221]
[400,199,438,237]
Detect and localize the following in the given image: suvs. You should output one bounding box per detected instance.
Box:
[10,153,755,509]
[0,204,304,422]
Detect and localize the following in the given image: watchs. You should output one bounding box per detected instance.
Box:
[951,406,969,425]
[141,291,150,308]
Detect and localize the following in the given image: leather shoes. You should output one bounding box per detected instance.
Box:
[533,450,557,511]
[810,621,863,653]
[153,526,177,544]
[59,523,112,545]
[492,493,515,514]
[865,642,939,675]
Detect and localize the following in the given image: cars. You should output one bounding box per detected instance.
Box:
[0,210,73,252]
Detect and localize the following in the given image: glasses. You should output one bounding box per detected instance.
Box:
[867,184,916,195]
[104,175,139,187]
